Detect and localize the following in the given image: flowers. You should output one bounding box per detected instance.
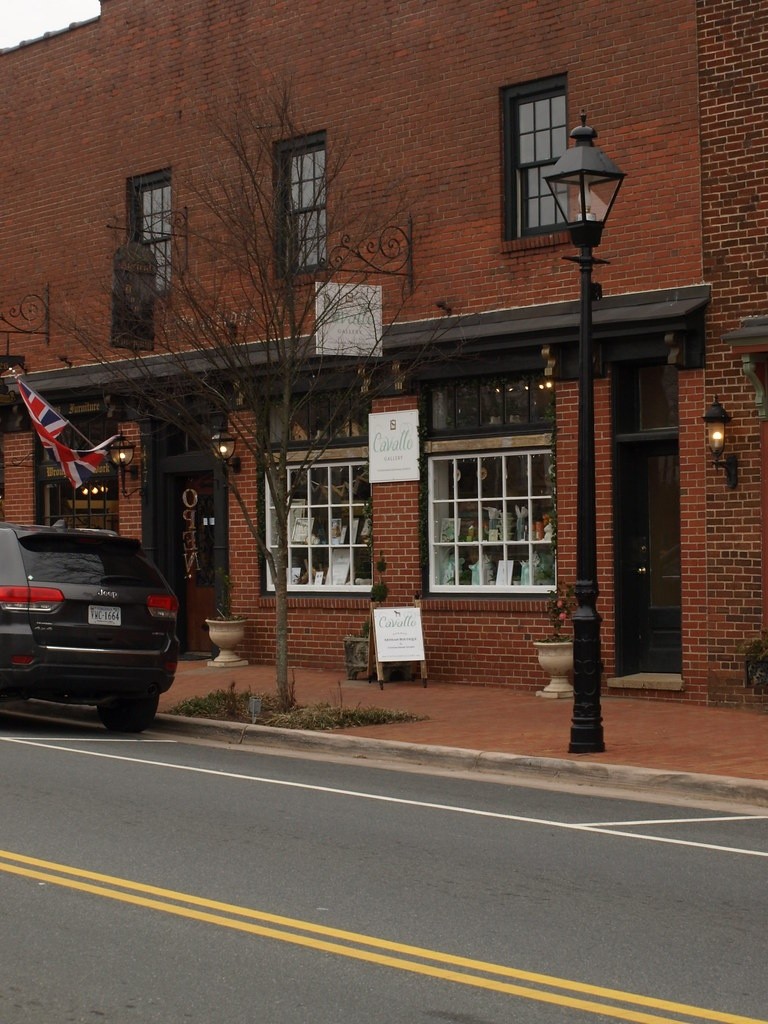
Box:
[533,586,575,642]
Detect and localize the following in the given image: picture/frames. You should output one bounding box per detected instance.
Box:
[326,549,350,584]
[440,519,461,541]
[292,518,313,542]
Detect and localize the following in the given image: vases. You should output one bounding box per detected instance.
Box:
[534,643,573,698]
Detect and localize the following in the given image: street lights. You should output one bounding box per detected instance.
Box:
[543,109,630,757]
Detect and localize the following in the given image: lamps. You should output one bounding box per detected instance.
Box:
[225,323,238,338]
[59,355,71,367]
[207,419,241,477]
[80,430,137,495]
[700,395,739,489]
[12,360,27,372]
[435,301,451,313]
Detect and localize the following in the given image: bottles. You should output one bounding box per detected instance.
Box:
[468,525,474,539]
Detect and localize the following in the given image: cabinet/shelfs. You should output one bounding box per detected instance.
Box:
[425,448,558,593]
[266,460,372,592]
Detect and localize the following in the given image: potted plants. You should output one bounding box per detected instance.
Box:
[343,551,411,682]
[204,573,248,669]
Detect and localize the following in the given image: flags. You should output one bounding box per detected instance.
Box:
[9,367,121,490]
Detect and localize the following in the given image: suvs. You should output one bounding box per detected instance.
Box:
[0,523,181,733]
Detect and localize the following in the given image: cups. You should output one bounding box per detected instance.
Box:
[536,521,543,539]
